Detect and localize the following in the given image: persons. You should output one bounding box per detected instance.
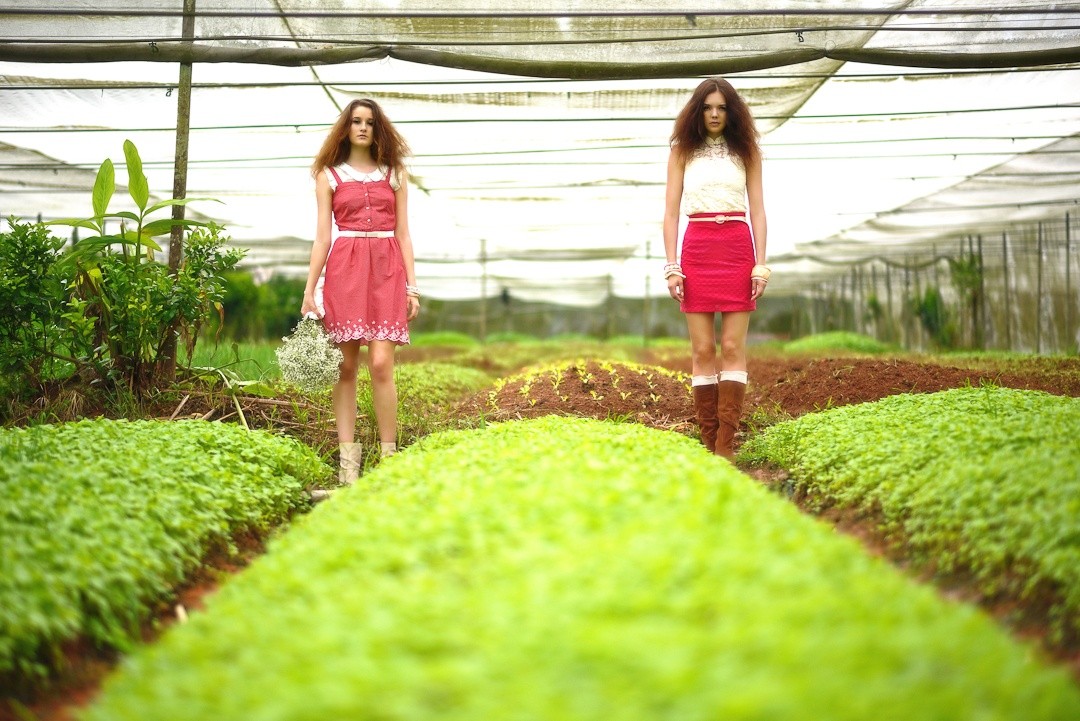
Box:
[300,98,420,486]
[664,77,770,463]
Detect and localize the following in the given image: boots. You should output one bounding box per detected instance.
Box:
[714,370,747,464]
[691,374,720,452]
[380,442,397,460]
[338,443,362,486]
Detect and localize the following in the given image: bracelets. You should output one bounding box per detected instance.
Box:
[405,286,421,297]
[751,264,770,282]
[664,263,686,279]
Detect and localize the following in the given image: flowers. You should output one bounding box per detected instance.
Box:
[270,275,344,393]
